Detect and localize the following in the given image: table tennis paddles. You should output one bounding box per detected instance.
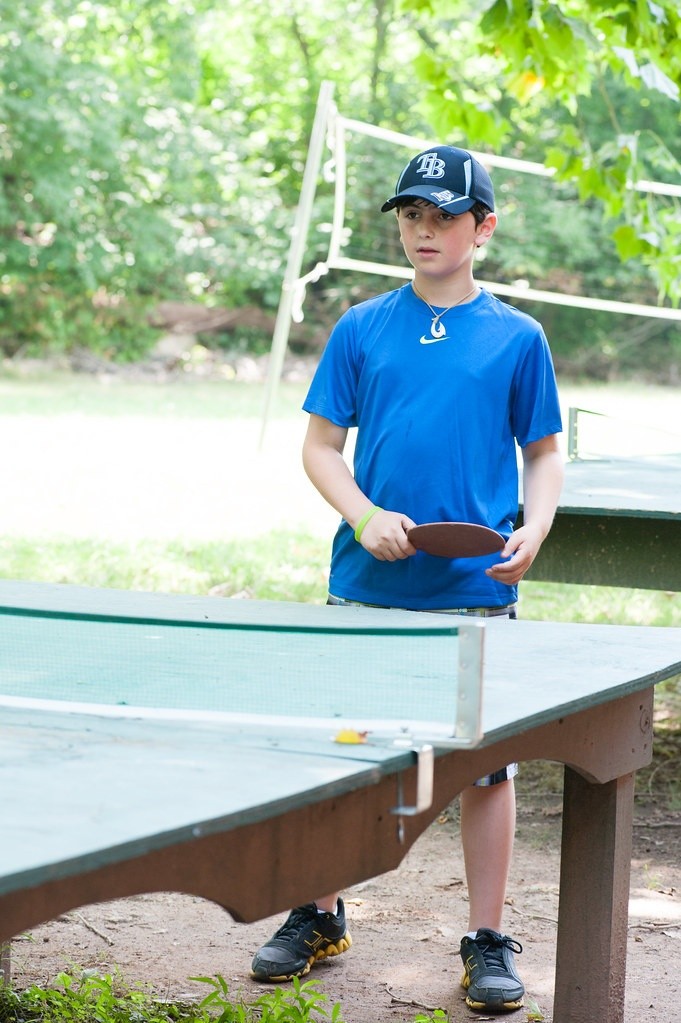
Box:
[408,521,506,557]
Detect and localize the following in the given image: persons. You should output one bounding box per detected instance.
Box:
[248,144,569,1012]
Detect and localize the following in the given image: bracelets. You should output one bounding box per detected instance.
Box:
[353,505,380,544]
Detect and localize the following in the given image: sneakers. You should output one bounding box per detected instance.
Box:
[460,927,525,1011]
[249,897,349,981]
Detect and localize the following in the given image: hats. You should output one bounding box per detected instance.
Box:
[381,147,496,215]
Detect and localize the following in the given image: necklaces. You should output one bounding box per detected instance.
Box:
[412,278,478,339]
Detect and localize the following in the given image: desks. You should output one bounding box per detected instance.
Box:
[0,579,681,1023]
